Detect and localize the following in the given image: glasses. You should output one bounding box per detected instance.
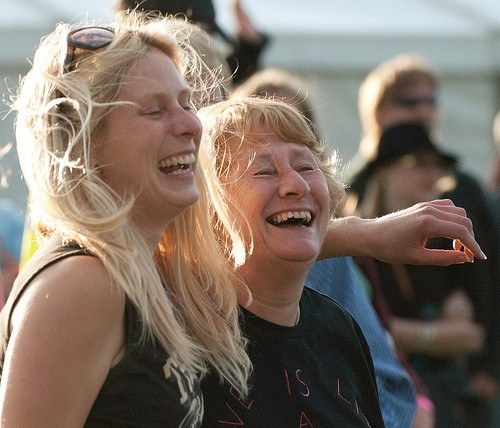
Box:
[59,27,115,98]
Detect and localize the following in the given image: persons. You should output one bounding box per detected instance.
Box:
[117,0,273,91]
[0,15,487,427]
[195,99,386,428]
[230,71,417,427]
[336,53,500,428]
[345,119,491,428]
